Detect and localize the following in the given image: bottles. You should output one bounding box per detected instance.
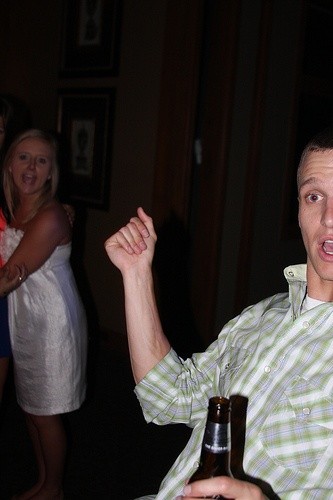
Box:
[186,396,234,500]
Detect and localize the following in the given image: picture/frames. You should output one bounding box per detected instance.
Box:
[57,0,124,80]
[54,84,117,211]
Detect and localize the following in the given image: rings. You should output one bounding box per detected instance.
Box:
[19,276,23,280]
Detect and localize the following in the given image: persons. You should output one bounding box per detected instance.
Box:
[0,128,89,500]
[0,96,76,402]
[103,132,332,500]
